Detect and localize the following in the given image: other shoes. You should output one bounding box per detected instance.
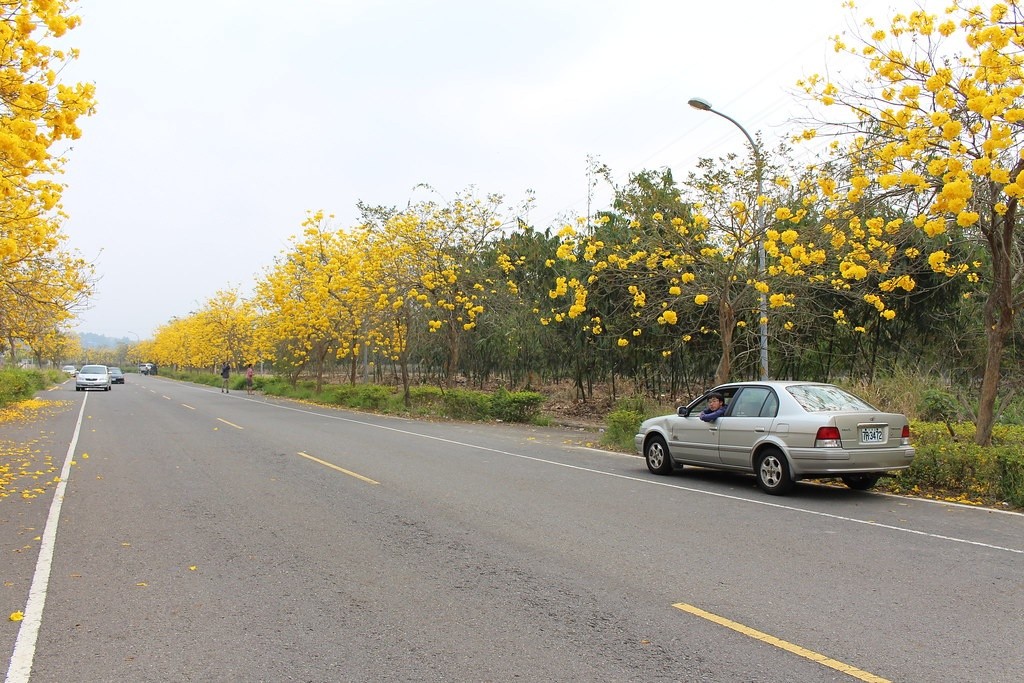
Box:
[251,393,254,395]
[227,391,229,393]
[248,393,250,394]
[222,390,223,392]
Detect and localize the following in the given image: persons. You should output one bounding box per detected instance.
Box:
[700,393,728,422]
[246,364,255,395]
[221,361,231,393]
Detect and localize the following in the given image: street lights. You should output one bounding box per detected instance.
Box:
[128,330,140,373]
[688,97,769,381]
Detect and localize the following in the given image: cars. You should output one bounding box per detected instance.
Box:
[139,363,153,372]
[108,367,124,384]
[62,366,77,377]
[634,380,915,497]
[75,365,112,391]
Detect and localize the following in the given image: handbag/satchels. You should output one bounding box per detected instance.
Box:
[221,373,223,375]
